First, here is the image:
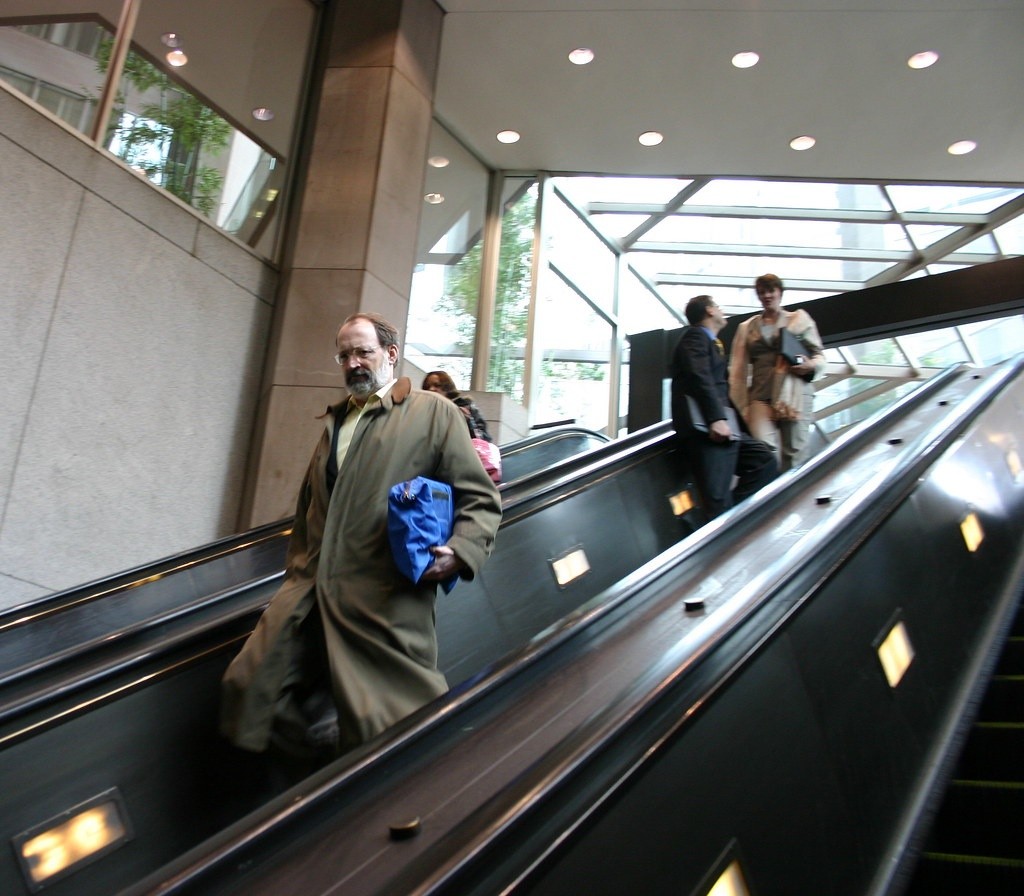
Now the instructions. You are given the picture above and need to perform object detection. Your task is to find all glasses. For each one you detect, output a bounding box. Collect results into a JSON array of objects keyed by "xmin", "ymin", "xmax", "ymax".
[
  {"xmin": 712, "ymin": 304, "xmax": 720, "ymax": 308},
  {"xmin": 335, "ymin": 345, "xmax": 382, "ymax": 366}
]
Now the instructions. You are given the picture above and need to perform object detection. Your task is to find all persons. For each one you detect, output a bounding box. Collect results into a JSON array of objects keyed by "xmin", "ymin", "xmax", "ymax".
[
  {"xmin": 223, "ymin": 314, "xmax": 503, "ymax": 873},
  {"xmin": 671, "ymin": 295, "xmax": 779, "ymax": 521},
  {"xmin": 728, "ymin": 274, "xmax": 825, "ymax": 476}
]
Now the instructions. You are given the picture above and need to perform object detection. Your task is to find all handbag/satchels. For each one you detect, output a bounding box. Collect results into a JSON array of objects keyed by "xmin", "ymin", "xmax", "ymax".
[
  {"xmin": 472, "ymin": 438, "xmax": 502, "ymax": 482},
  {"xmin": 386, "ymin": 475, "xmax": 463, "ymax": 596}
]
